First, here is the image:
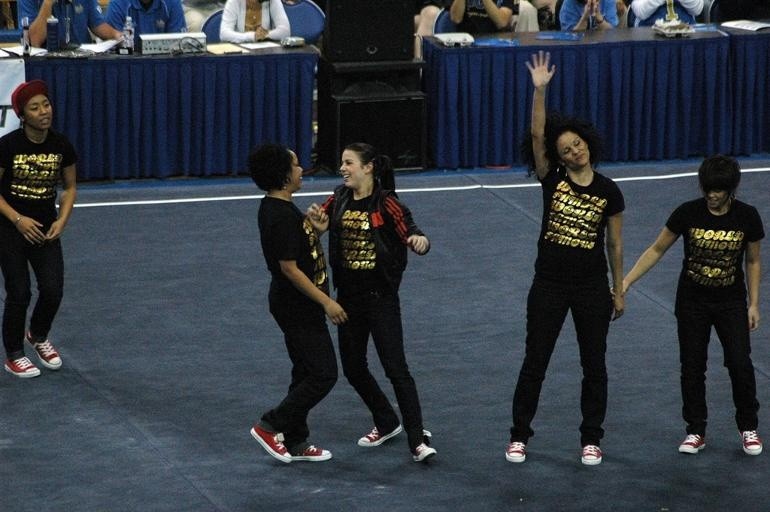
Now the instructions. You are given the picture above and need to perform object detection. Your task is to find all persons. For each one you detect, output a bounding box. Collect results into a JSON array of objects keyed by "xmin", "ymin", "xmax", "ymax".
[
  {"xmin": 0, "ymin": 80, "xmax": 79, "ymax": 380},
  {"xmin": 16, "ymin": 2, "xmax": 705, "ymax": 55},
  {"xmin": 248, "ymin": 142, "xmax": 348, "ymax": 464},
  {"xmin": 303, "ymin": 142, "xmax": 440, "ymax": 464},
  {"xmin": 608, "ymin": 153, "xmax": 765, "ymax": 457},
  {"xmin": 505, "ymin": 50, "xmax": 627, "ymax": 466}
]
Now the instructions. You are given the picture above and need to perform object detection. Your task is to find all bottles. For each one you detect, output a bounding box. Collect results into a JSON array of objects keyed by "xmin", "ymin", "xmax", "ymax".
[
  {"xmin": 123, "ymin": 15, "xmax": 136, "ymax": 53},
  {"xmin": 45, "ymin": 14, "xmax": 59, "ymax": 53}
]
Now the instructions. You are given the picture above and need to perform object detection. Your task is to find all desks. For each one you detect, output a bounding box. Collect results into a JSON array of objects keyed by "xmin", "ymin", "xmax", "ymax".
[
  {"xmin": 423, "ymin": 25, "xmax": 732, "ymax": 170},
  {"xmin": 723, "ymin": 18, "xmax": 770, "ymax": 156},
  {"xmin": 23, "ymin": 40, "xmax": 321, "ymax": 181}
]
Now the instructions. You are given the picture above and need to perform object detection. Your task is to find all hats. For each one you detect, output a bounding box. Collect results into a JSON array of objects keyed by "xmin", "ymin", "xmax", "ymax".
[{"xmin": 11, "ymin": 80, "xmax": 48, "ymax": 116}]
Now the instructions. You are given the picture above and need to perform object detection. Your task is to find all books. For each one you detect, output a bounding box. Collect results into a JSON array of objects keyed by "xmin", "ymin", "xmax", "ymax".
[{"xmin": 719, "ymin": 18, "xmax": 770, "ymax": 33}]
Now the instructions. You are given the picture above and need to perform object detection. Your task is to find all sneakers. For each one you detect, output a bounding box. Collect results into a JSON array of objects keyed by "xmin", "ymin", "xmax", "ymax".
[
  {"xmin": 4, "ymin": 357, "xmax": 41, "ymax": 378},
  {"xmin": 25, "ymin": 332, "xmax": 62, "ymax": 370},
  {"xmin": 679, "ymin": 433, "xmax": 705, "ymax": 454},
  {"xmin": 357, "ymin": 425, "xmax": 402, "ymax": 447},
  {"xmin": 249, "ymin": 427, "xmax": 293, "ymax": 464},
  {"xmin": 738, "ymin": 429, "xmax": 762, "ymax": 456},
  {"xmin": 505, "ymin": 441, "xmax": 525, "ymax": 462},
  {"xmin": 291, "ymin": 445, "xmax": 332, "ymax": 462},
  {"xmin": 412, "ymin": 443, "xmax": 437, "ymax": 461},
  {"xmin": 581, "ymin": 445, "xmax": 602, "ymax": 464}
]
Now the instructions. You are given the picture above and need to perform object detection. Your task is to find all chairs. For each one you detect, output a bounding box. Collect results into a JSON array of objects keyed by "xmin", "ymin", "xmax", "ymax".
[
  {"xmin": 413, "ymin": 4, "xmax": 442, "ymax": 78},
  {"xmin": 201, "ymin": 9, "xmax": 224, "ymax": 44},
  {"xmin": 279, "ymin": 1, "xmax": 330, "ymax": 44}
]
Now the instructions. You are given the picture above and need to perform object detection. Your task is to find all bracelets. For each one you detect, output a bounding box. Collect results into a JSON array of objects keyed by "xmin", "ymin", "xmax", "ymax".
[{"xmin": 13, "ymin": 215, "xmax": 23, "ymax": 226}]
